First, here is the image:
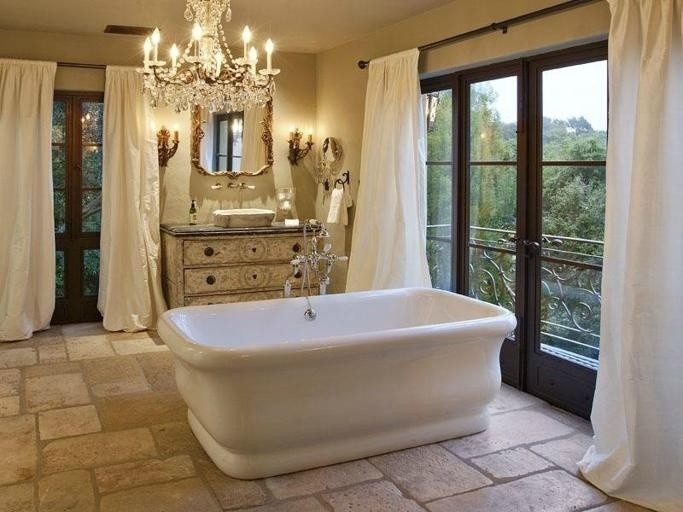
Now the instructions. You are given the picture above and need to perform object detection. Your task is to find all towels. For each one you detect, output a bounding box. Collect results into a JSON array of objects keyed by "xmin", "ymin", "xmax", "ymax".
[
  {"xmin": 326, "ymin": 188, "xmax": 349, "ymax": 227},
  {"xmin": 343, "ymin": 183, "xmax": 353, "ymax": 208}
]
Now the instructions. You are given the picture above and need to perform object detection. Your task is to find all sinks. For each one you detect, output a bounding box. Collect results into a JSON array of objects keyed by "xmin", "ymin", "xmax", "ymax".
[{"xmin": 213, "ymin": 208, "xmax": 276, "ymax": 228}]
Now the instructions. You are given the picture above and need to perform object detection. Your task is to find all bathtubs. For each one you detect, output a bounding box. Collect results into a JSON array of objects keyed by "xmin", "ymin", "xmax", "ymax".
[{"xmin": 156, "ymin": 287, "xmax": 518, "ymax": 480}]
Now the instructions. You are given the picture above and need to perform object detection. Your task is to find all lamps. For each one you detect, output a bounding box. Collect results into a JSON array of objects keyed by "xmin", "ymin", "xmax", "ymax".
[
  {"xmin": 136, "ymin": 1, "xmax": 281, "ymax": 114},
  {"xmin": 287, "ymin": 128, "xmax": 314, "ymax": 166},
  {"xmin": 155, "ymin": 124, "xmax": 179, "ymax": 167},
  {"xmin": 426, "ymin": 91, "xmax": 440, "ymax": 133}
]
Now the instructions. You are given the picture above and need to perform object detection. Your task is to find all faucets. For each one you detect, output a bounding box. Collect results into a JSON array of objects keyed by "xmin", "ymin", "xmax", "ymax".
[{"xmin": 230, "ymin": 182, "xmax": 247, "ymax": 189}]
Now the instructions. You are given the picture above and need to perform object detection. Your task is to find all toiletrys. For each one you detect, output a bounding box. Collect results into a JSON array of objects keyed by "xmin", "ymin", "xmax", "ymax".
[{"xmin": 189, "ymin": 199, "xmax": 197, "ymax": 225}]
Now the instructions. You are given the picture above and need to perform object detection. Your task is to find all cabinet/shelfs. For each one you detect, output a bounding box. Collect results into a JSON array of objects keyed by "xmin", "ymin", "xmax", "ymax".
[{"xmin": 158, "ymin": 224, "xmax": 324, "ymax": 311}]
